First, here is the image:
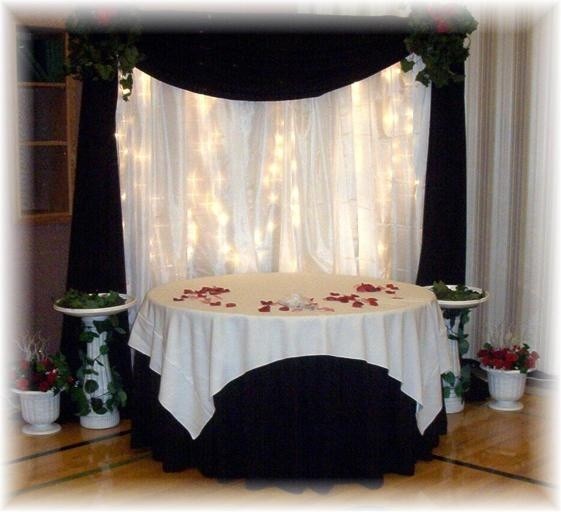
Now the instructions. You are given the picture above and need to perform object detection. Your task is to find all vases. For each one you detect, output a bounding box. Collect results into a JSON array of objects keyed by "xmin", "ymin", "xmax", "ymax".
[
  {"xmin": 12, "ymin": 389, "xmax": 65, "ymax": 435},
  {"xmin": 480, "ymin": 363, "xmax": 536, "ymax": 413}
]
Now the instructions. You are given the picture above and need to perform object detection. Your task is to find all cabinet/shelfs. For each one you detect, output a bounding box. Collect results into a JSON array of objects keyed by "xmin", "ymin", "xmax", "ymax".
[{"xmin": 8, "ymin": 17, "xmax": 77, "ymax": 224}]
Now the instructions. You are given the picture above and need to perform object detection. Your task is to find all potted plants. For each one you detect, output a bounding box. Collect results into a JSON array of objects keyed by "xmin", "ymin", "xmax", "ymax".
[
  {"xmin": 423, "ymin": 281, "xmax": 489, "ymax": 415},
  {"xmin": 51, "ymin": 288, "xmax": 136, "ymax": 430}
]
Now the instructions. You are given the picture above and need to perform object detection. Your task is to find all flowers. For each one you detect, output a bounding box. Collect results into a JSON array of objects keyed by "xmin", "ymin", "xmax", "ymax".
[
  {"xmin": 8, "ymin": 328, "xmax": 76, "ymax": 396},
  {"xmin": 474, "ymin": 338, "xmax": 539, "ymax": 377},
  {"xmin": 400, "ymin": 1, "xmax": 477, "ymax": 86},
  {"xmin": 65, "ymin": 4, "xmax": 144, "ymax": 102}
]
